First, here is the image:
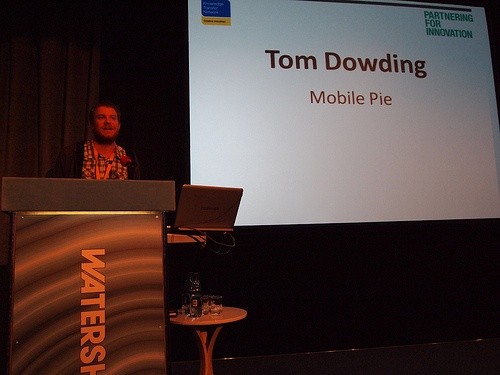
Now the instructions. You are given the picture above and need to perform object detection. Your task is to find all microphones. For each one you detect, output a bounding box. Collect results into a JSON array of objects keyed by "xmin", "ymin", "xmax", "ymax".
[{"xmin": 120, "ymin": 155, "xmax": 136, "ymax": 169}]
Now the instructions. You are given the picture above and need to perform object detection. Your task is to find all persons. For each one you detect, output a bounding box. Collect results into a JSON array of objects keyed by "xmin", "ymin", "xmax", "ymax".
[{"xmin": 47, "ymin": 99, "xmax": 154, "ymax": 180}]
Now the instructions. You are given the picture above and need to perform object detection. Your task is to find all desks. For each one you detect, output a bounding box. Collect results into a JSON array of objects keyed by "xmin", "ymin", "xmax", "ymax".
[{"xmin": 170, "ymin": 307, "xmax": 248, "ymax": 375}]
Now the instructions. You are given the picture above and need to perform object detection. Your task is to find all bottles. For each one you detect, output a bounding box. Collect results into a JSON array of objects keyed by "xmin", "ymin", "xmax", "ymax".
[
  {"xmin": 185, "ymin": 272, "xmax": 193, "ymax": 316},
  {"xmin": 189, "ymin": 272, "xmax": 202, "ymax": 318}
]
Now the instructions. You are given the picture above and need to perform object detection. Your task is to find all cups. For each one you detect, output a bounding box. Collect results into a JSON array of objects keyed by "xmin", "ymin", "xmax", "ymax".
[
  {"xmin": 211, "ymin": 295, "xmax": 223, "ymax": 314},
  {"xmin": 201, "ymin": 296, "xmax": 210, "ymax": 315}
]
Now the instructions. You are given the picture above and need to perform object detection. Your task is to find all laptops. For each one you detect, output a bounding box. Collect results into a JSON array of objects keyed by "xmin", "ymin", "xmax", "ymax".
[{"xmin": 166, "ymin": 184, "xmax": 243, "ymax": 231}]
